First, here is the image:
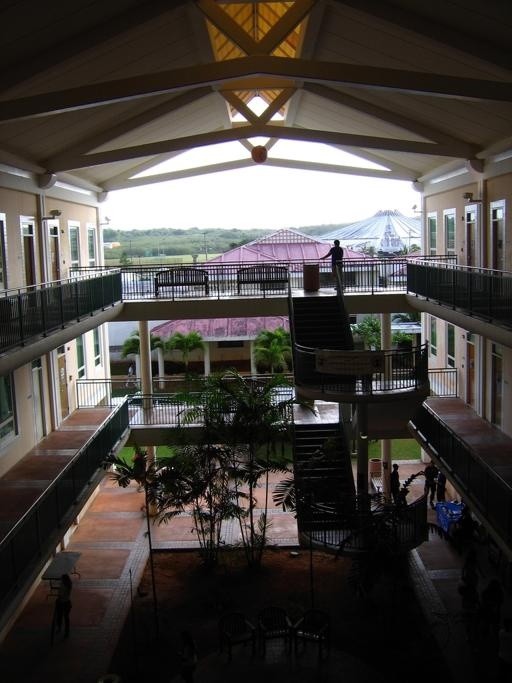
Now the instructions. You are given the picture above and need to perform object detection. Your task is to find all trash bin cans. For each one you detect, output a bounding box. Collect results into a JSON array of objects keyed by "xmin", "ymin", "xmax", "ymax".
[
  {"xmin": 370, "ymin": 459, "xmax": 381, "ymax": 477},
  {"xmin": 303, "ymin": 264, "xmax": 319, "ymax": 291}
]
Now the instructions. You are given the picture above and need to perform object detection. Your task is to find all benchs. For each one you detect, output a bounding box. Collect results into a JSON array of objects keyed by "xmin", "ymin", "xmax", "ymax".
[
  {"xmin": 154, "ymin": 266, "xmax": 291, "ymax": 295},
  {"xmin": 371, "ymin": 471, "xmax": 383, "ymax": 493}
]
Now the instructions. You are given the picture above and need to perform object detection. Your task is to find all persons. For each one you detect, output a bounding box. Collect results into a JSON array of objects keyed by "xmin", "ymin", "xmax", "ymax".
[
  {"xmin": 56, "ymin": 573, "xmax": 71, "ymax": 636},
  {"xmin": 456, "ymin": 564, "xmax": 479, "ymax": 655},
  {"xmin": 434, "ymin": 470, "xmax": 446, "ymax": 501},
  {"xmin": 479, "ymin": 575, "xmax": 505, "ymax": 640},
  {"xmin": 424, "ymin": 459, "xmax": 436, "ymax": 507},
  {"xmin": 125, "ymin": 361, "xmax": 137, "ymax": 387},
  {"xmin": 391, "ymin": 463, "xmax": 401, "ymax": 506},
  {"xmin": 319, "ymin": 239, "xmax": 344, "ymax": 289}
]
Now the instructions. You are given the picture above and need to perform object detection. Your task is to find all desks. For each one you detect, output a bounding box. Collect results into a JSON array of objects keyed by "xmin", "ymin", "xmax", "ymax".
[
  {"xmin": 43, "ymin": 550, "xmax": 84, "ymax": 599},
  {"xmin": 435, "ymin": 502, "xmax": 471, "ymax": 541}
]
